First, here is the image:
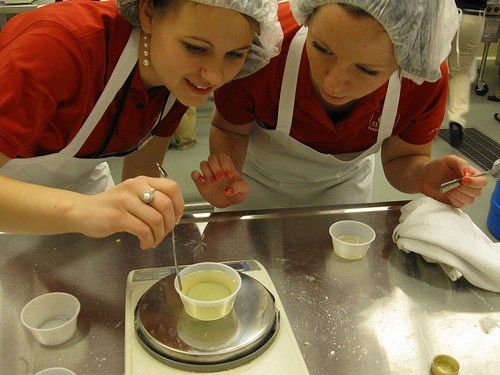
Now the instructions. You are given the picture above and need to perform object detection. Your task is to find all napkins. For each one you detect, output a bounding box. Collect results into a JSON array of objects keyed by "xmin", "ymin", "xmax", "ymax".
[{"xmin": 392, "ymin": 195, "xmax": 500, "ymax": 294}]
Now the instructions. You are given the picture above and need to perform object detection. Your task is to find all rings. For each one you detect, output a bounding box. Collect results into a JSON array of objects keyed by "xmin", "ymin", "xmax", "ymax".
[{"xmin": 142, "ymin": 188, "xmax": 156, "ymax": 203}]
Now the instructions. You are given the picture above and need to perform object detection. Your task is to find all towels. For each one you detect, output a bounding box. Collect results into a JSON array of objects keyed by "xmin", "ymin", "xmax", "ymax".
[{"xmin": 390, "ymin": 195, "xmax": 500, "ymax": 294}]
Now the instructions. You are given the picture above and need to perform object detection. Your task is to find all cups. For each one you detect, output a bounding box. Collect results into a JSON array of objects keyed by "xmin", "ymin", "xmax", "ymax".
[
  {"xmin": 20, "ymin": 291, "xmax": 81, "ymax": 346},
  {"xmin": 328, "ymin": 220, "xmax": 376, "ymax": 259},
  {"xmin": 174, "ymin": 262, "xmax": 243, "ymax": 320}
]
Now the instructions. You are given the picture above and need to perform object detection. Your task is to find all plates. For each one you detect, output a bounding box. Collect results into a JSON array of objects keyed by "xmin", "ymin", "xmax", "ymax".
[{"xmin": 33, "ymin": 366, "xmax": 76, "ymax": 375}]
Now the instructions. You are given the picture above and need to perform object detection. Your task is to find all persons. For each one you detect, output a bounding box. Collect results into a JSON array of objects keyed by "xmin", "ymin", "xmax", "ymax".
[
  {"xmin": 188, "ymin": 0, "xmax": 486, "ymax": 208},
  {"xmin": 0, "ymin": 0, "xmax": 262, "ymax": 251}
]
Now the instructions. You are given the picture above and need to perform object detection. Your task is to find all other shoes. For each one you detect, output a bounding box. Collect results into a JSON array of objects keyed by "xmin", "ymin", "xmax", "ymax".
[
  {"xmin": 449, "ymin": 122, "xmax": 463, "ymax": 147},
  {"xmin": 488, "ymin": 95, "xmax": 500, "ymax": 102}
]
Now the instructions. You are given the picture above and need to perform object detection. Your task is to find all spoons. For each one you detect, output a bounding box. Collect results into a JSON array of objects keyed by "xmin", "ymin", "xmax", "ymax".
[{"xmin": 439, "ymin": 158, "xmax": 500, "ymax": 195}]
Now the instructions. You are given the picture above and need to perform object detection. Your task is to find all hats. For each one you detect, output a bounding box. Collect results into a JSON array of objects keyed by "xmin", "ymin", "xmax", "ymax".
[
  {"xmin": 115, "ymin": 0, "xmax": 284, "ymax": 80},
  {"xmin": 288, "ymin": 0, "xmax": 459, "ymax": 85}
]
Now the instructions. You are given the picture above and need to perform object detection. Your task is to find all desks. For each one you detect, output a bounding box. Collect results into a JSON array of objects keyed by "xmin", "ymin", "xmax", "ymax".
[{"xmin": 0, "ymin": 200, "xmax": 500, "ymax": 375}]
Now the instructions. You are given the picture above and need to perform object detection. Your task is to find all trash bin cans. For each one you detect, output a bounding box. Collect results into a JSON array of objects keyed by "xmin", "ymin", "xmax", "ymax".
[{"xmin": 486, "ymin": 180, "xmax": 500, "ymax": 240}]
